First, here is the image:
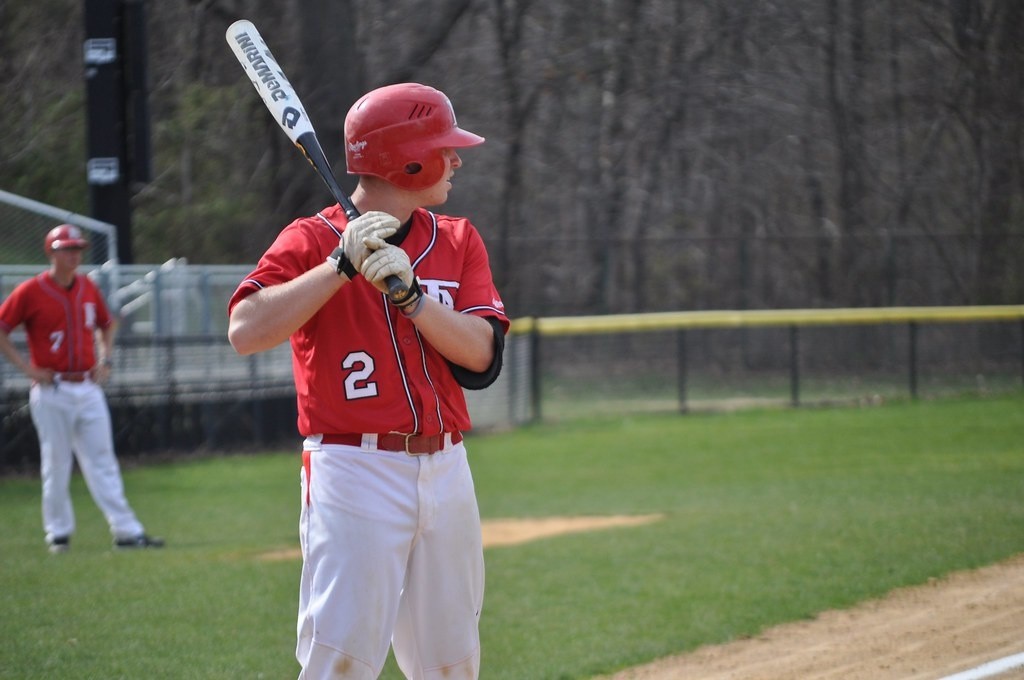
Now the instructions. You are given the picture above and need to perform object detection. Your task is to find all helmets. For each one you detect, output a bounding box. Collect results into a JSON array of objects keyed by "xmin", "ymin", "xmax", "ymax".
[
  {"xmin": 44, "ymin": 224, "xmax": 89, "ymax": 254},
  {"xmin": 344, "ymin": 82, "xmax": 485, "ymax": 192}
]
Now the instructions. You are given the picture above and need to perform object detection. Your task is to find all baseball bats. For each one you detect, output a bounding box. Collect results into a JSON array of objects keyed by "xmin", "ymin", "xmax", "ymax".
[{"xmin": 224, "ymin": 19, "xmax": 410, "ymax": 303}]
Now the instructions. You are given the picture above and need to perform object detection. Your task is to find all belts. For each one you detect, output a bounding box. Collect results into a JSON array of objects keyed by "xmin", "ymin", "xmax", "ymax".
[
  {"xmin": 321, "ymin": 430, "xmax": 463, "ymax": 456},
  {"xmin": 61, "ymin": 371, "xmax": 85, "ymax": 383}
]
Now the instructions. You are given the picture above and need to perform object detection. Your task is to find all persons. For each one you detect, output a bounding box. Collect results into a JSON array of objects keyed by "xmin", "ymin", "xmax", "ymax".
[
  {"xmin": 0, "ymin": 224, "xmax": 164, "ymax": 549},
  {"xmin": 229, "ymin": 83, "xmax": 510, "ymax": 680}
]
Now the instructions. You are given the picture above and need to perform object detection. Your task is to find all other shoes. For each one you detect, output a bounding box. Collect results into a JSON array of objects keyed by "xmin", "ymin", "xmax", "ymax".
[
  {"xmin": 49, "ymin": 535, "xmax": 69, "ymax": 552},
  {"xmin": 115, "ymin": 532, "xmax": 164, "ymax": 547}
]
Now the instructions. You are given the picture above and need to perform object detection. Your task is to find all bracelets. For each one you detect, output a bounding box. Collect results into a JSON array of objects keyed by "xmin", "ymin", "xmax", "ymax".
[
  {"xmin": 99, "ymin": 358, "xmax": 112, "ymax": 366},
  {"xmin": 400, "ymin": 294, "xmax": 425, "ymax": 318}
]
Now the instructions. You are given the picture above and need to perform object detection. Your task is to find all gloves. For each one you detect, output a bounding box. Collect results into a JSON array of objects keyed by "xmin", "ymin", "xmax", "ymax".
[
  {"xmin": 360, "ymin": 237, "xmax": 425, "ymax": 318},
  {"xmin": 326, "ymin": 210, "xmax": 400, "ymax": 282}
]
